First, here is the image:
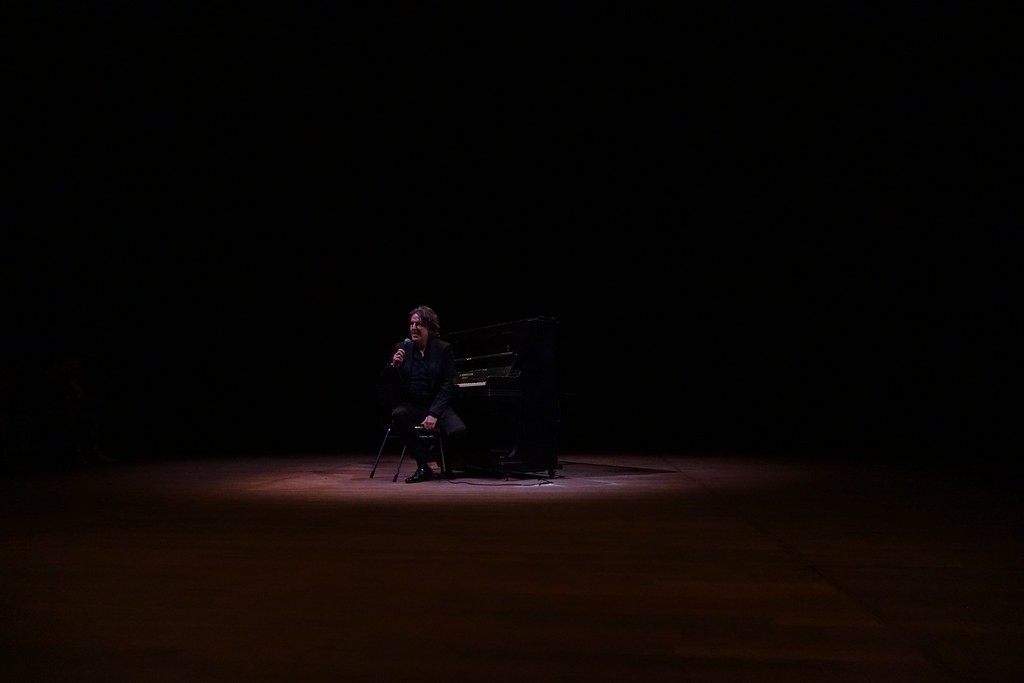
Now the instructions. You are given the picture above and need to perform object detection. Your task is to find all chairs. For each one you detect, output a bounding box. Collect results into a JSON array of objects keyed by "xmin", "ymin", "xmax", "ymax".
[{"xmin": 369, "ymin": 420, "xmax": 447, "ymax": 484}]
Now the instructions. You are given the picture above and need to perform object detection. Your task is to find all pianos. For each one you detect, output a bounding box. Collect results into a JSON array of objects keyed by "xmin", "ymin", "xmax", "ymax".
[{"xmin": 394, "ymin": 314, "xmax": 563, "ymax": 478}]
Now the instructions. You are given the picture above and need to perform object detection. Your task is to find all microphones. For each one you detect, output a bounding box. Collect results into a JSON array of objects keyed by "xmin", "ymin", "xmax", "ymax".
[{"xmin": 393, "ymin": 338, "xmax": 411, "ymax": 367}]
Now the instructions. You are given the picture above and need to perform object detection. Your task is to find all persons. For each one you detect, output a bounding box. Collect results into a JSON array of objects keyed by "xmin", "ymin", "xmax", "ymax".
[{"xmin": 378, "ymin": 306, "xmax": 467, "ymax": 483}]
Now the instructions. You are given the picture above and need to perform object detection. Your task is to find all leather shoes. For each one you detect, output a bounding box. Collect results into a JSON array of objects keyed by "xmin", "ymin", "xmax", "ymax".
[{"xmin": 405, "ymin": 468, "xmax": 433, "ymax": 483}]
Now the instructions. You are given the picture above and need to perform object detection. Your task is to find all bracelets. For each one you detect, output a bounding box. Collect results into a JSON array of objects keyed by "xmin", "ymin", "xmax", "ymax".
[{"xmin": 391, "ymin": 362, "xmax": 400, "ymax": 368}]
